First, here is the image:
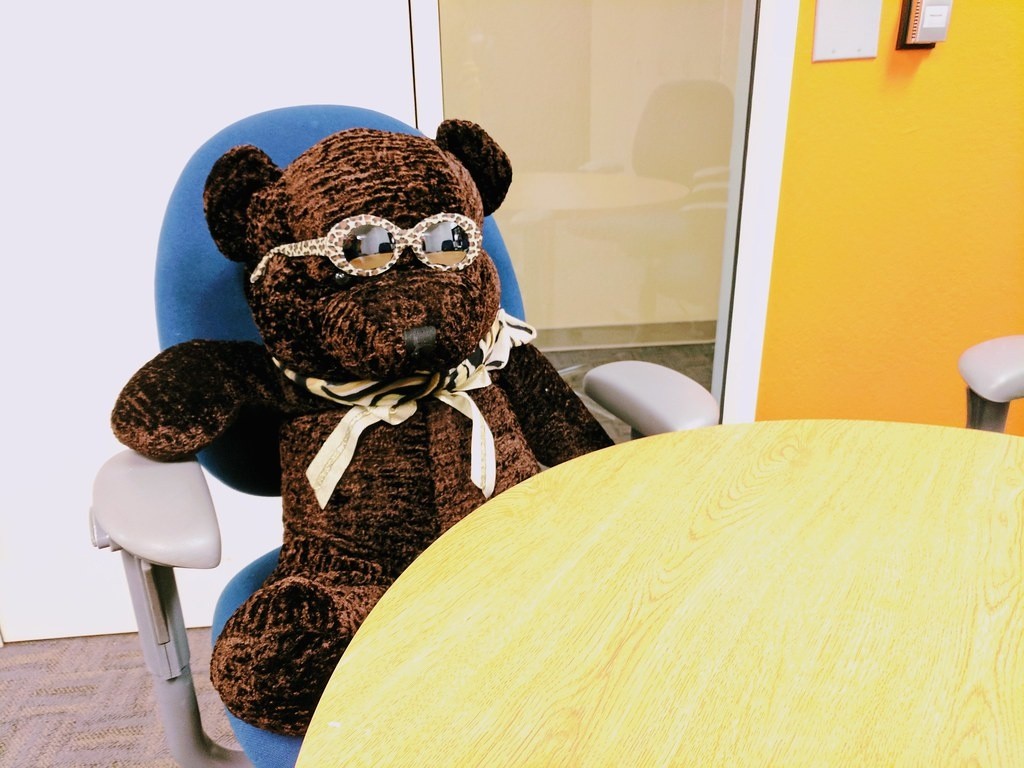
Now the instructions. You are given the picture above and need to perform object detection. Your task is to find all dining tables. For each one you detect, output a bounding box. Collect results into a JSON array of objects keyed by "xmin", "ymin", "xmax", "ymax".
[{"xmin": 295, "ymin": 418, "xmax": 1024, "ymax": 768}]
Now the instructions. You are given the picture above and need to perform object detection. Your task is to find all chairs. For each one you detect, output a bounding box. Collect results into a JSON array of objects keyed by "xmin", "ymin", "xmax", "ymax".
[{"xmin": 85, "ymin": 97, "xmax": 722, "ymax": 768}]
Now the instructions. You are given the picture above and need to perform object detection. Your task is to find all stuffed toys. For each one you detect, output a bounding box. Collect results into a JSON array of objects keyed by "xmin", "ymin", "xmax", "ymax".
[{"xmin": 108, "ymin": 118, "xmax": 616, "ymax": 740}]
